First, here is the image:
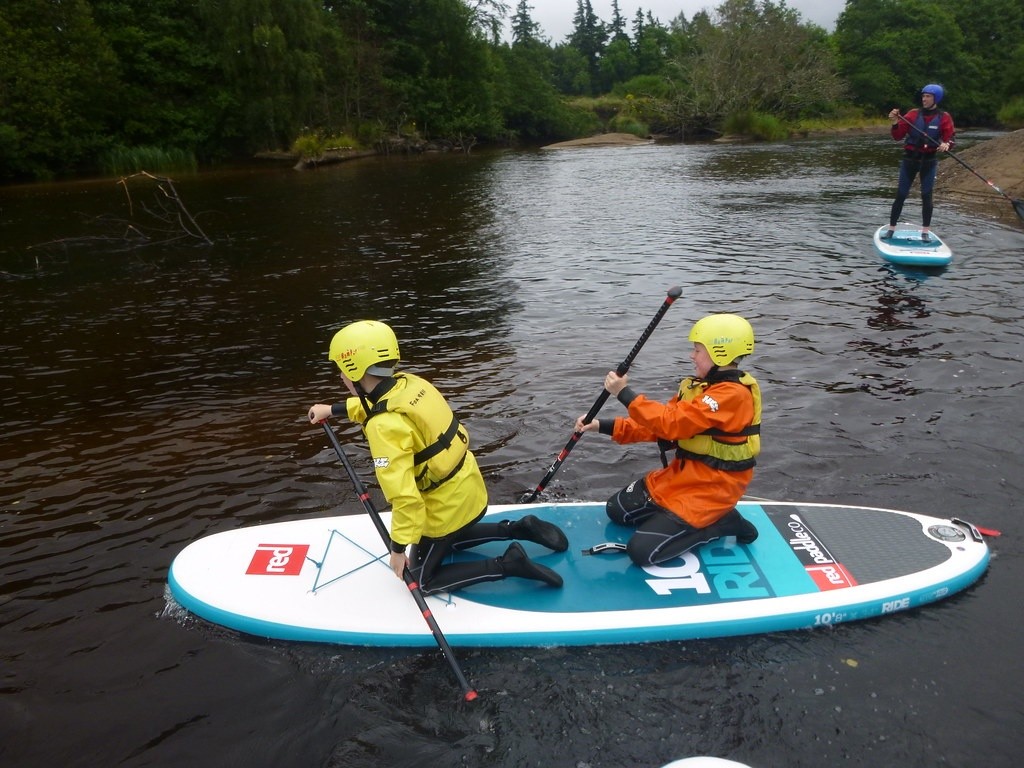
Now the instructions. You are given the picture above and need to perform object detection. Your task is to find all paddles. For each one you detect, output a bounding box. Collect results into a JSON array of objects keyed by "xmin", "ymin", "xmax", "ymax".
[
  {"xmin": 516, "ymin": 287, "xmax": 682, "ymax": 504},
  {"xmin": 892, "ymin": 109, "xmax": 1024, "ymax": 221},
  {"xmin": 312, "ymin": 408, "xmax": 506, "ymax": 749}
]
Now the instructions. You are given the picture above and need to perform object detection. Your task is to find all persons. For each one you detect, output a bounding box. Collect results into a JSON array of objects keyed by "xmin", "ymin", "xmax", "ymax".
[
  {"xmin": 308, "ymin": 320, "xmax": 569, "ymax": 597},
  {"xmin": 573, "ymin": 314, "xmax": 761, "ymax": 566},
  {"xmin": 880, "ymin": 84, "xmax": 955, "ymax": 243}
]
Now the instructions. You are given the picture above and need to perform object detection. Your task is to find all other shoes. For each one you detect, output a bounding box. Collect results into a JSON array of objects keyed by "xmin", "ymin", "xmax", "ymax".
[
  {"xmin": 495, "ymin": 542, "xmax": 563, "ymax": 587},
  {"xmin": 921, "ymin": 233, "xmax": 931, "ymax": 242},
  {"xmin": 881, "ymin": 230, "xmax": 894, "ymax": 239},
  {"xmin": 717, "ymin": 508, "xmax": 758, "ymax": 544},
  {"xmin": 498, "ymin": 515, "xmax": 568, "ymax": 552}
]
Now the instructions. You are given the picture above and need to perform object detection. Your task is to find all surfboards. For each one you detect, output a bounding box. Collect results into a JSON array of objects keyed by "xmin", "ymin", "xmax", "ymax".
[
  {"xmin": 167, "ymin": 499, "xmax": 988, "ymax": 651},
  {"xmin": 870, "ymin": 222, "xmax": 951, "ymax": 268}
]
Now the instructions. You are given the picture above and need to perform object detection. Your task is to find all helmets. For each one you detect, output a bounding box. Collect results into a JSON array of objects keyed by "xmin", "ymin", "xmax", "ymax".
[
  {"xmin": 920, "ymin": 84, "xmax": 943, "ymax": 104},
  {"xmin": 689, "ymin": 314, "xmax": 754, "ymax": 367},
  {"xmin": 329, "ymin": 320, "xmax": 401, "ymax": 382}
]
